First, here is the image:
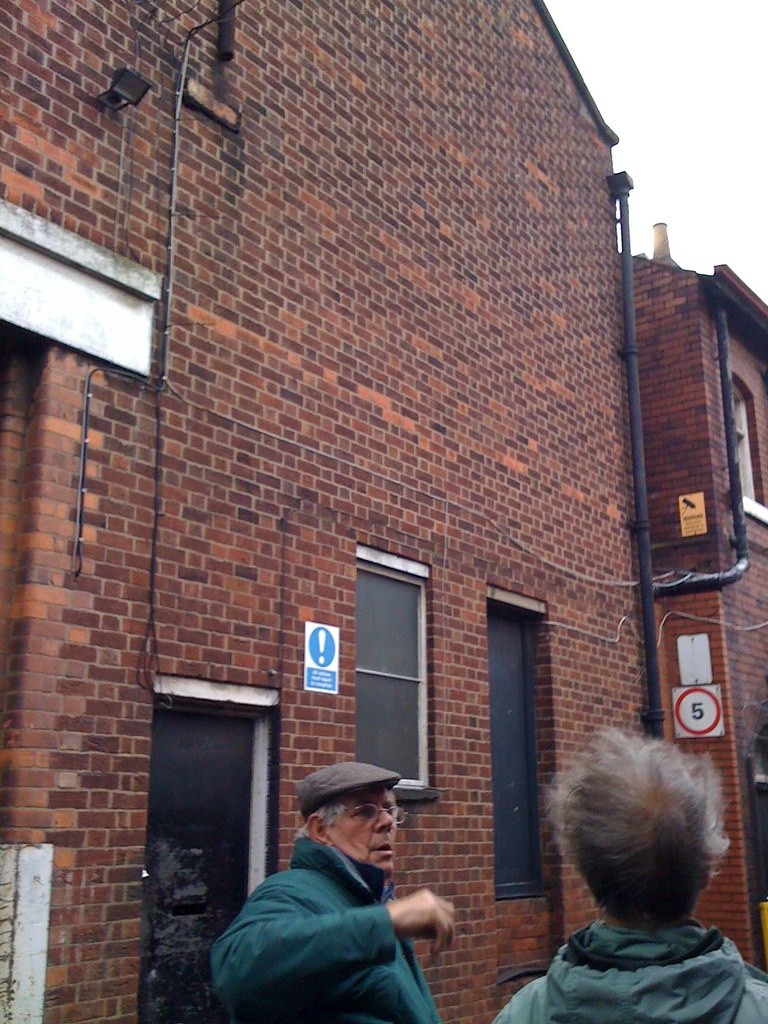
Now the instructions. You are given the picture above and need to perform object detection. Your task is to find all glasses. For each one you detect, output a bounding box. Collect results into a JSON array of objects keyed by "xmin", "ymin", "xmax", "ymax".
[{"xmin": 324, "ymin": 802, "xmax": 409, "ymax": 823}]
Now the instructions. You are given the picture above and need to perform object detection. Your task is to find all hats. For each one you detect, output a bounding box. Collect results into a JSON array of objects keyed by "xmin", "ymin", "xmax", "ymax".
[{"xmin": 298, "ymin": 762, "xmax": 402, "ymax": 822}]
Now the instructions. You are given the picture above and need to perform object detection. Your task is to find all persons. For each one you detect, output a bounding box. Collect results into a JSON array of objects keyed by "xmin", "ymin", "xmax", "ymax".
[
  {"xmin": 206, "ymin": 759, "xmax": 456, "ymax": 1024},
  {"xmin": 487, "ymin": 727, "xmax": 768, "ymax": 1023}
]
